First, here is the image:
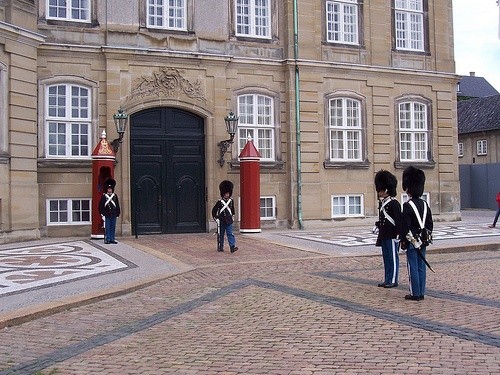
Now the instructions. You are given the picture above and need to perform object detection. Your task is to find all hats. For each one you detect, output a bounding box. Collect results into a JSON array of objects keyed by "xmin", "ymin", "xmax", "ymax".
[
  {"xmin": 402, "ymin": 166, "xmax": 425, "ymax": 197},
  {"xmin": 104, "ymin": 178, "xmax": 116, "ymax": 190},
  {"xmin": 219, "ymin": 180, "xmax": 233, "ymax": 197},
  {"xmin": 375, "ymin": 170, "xmax": 397, "ymax": 196}
]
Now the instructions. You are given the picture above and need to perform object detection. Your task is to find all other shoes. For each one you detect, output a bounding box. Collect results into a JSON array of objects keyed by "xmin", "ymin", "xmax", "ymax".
[
  {"xmin": 384, "ymin": 283, "xmax": 398, "ymax": 288},
  {"xmin": 110, "ymin": 240, "xmax": 117, "ymax": 244},
  {"xmin": 231, "ymin": 246, "xmax": 238, "ymax": 253},
  {"xmin": 220, "ymin": 248, "xmax": 224, "ymax": 252},
  {"xmin": 405, "ymin": 294, "xmax": 424, "ymax": 300},
  {"xmin": 378, "ymin": 282, "xmax": 387, "ymax": 287},
  {"xmin": 489, "ymin": 225, "xmax": 495, "ymax": 228},
  {"xmin": 105, "ymin": 241, "xmax": 109, "ymax": 244}
]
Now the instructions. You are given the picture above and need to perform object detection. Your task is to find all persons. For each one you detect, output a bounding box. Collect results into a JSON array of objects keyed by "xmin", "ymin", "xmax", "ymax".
[
  {"xmin": 372, "ymin": 170, "xmax": 402, "ymax": 288},
  {"xmin": 212, "ymin": 180, "xmax": 238, "ymax": 253},
  {"xmin": 401, "ymin": 168, "xmax": 434, "ymax": 302},
  {"xmin": 489, "ymin": 192, "xmax": 500, "ymax": 228},
  {"xmin": 97, "ymin": 178, "xmax": 120, "ymax": 244}
]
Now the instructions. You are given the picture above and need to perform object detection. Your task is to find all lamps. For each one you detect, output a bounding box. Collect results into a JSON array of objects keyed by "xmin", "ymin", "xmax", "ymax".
[
  {"xmin": 216, "ymin": 110, "xmax": 239, "ymax": 168},
  {"xmin": 109, "ymin": 106, "xmax": 129, "ymax": 167}
]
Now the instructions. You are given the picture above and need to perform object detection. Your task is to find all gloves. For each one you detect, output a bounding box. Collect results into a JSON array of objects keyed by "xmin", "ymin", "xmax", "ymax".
[
  {"xmin": 216, "ymin": 219, "xmax": 219, "ymax": 223},
  {"xmin": 372, "ymin": 226, "xmax": 380, "ymax": 234}
]
